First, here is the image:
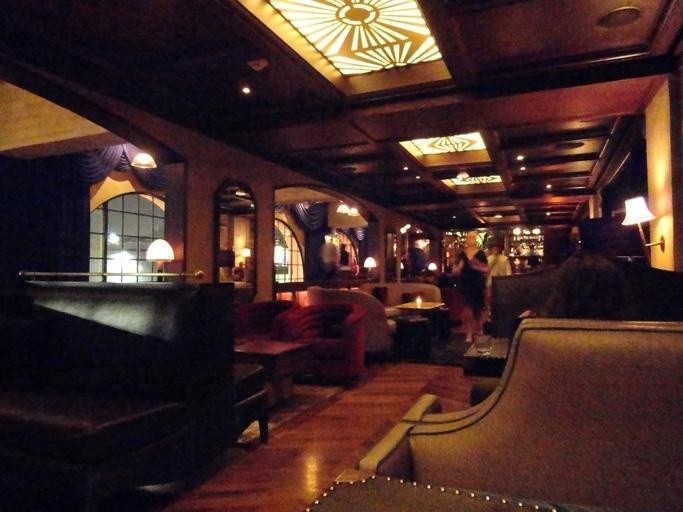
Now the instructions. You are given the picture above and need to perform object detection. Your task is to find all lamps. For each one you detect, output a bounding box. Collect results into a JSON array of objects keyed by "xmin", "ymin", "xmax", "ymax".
[
  {"xmin": 128, "ymin": 152, "xmax": 158, "ymax": 171},
  {"xmin": 620, "ymin": 196, "xmax": 665, "ymax": 252},
  {"xmin": 145, "ymin": 237, "xmax": 175, "ymax": 281}
]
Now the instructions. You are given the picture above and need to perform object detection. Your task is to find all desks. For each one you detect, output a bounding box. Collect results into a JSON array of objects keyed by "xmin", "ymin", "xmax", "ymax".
[{"xmin": 304, "ymin": 469, "xmax": 594, "ymax": 510}]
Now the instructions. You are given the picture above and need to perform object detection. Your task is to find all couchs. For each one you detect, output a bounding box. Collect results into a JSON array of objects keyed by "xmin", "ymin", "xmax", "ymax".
[{"xmin": 215, "ymin": 283, "xmax": 272, "ymax": 447}]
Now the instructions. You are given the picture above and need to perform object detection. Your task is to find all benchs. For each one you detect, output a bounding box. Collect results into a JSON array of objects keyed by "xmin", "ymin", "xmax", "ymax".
[
  {"xmin": 356, "ymin": 323, "xmax": 683, "ymax": 511},
  {"xmin": 236, "ymin": 260, "xmax": 680, "ymax": 423},
  {"xmin": 3, "ymin": 278, "xmax": 215, "ymax": 495}
]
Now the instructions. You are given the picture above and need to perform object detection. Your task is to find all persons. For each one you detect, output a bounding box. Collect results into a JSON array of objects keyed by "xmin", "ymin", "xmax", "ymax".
[
  {"xmin": 384, "ymin": 244, "xmax": 539, "ymax": 344},
  {"xmin": 562, "ymin": 234, "xmax": 634, "ymax": 321}
]
